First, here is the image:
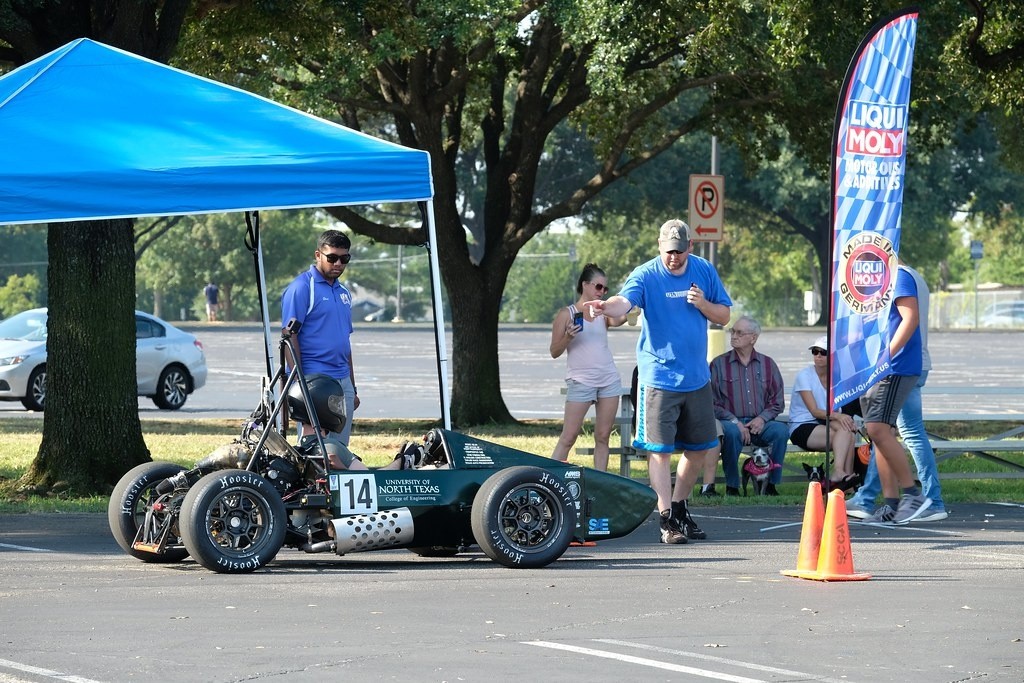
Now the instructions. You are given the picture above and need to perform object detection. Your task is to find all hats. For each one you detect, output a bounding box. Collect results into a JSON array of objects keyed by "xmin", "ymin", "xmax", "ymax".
[
  {"xmin": 659, "ymin": 220, "xmax": 692, "ymax": 253},
  {"xmin": 808, "ymin": 337, "xmax": 827, "ymax": 351}
]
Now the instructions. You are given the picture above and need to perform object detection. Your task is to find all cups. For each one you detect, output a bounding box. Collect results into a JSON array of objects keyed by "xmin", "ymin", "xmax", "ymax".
[{"xmin": 626, "ymin": 305, "xmax": 638, "ymax": 326}]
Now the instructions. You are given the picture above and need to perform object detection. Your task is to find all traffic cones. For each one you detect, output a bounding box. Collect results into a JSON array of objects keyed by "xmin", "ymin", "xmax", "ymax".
[
  {"xmin": 799, "ymin": 487, "xmax": 871, "ymax": 580},
  {"xmin": 782, "ymin": 482, "xmax": 828, "ymax": 579}
]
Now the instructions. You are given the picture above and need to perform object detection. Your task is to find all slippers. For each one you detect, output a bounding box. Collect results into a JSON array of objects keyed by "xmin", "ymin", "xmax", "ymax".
[{"xmin": 829, "ymin": 473, "xmax": 861, "ymax": 492}]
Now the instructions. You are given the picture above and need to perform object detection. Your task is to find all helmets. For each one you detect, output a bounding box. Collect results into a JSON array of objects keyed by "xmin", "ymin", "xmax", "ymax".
[{"xmin": 287, "ymin": 372, "xmax": 347, "ymax": 434}]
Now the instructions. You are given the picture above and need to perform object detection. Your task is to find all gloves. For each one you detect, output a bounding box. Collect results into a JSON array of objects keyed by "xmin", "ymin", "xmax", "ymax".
[{"xmin": 395, "ymin": 440, "xmax": 424, "ymax": 470}]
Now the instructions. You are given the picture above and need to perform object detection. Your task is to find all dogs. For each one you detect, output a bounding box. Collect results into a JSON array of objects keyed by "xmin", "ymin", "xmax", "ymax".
[
  {"xmin": 802, "ymin": 462, "xmax": 838, "ymax": 494},
  {"xmin": 741, "ymin": 443, "xmax": 774, "ymax": 496}
]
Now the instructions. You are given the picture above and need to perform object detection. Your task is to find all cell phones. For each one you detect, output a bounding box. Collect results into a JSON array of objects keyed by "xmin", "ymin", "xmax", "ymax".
[{"xmin": 574, "ymin": 312, "xmax": 583, "ymax": 331}]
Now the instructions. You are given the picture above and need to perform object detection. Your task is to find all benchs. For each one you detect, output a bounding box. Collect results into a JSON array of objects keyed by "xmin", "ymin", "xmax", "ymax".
[
  {"xmin": 624, "ymin": 442, "xmax": 866, "ymax": 454},
  {"xmin": 577, "ymin": 446, "xmax": 623, "ymax": 458}
]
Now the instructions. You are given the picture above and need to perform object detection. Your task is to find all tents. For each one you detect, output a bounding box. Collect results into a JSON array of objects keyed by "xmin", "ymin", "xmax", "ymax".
[{"xmin": 0, "ymin": 37, "xmax": 451, "ymax": 431}]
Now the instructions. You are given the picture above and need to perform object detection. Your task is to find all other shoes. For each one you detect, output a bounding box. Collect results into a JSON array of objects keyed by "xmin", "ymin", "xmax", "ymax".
[
  {"xmin": 726, "ymin": 486, "xmax": 741, "ymax": 496},
  {"xmin": 699, "ymin": 483, "xmax": 723, "ymax": 498},
  {"xmin": 767, "ymin": 484, "xmax": 780, "ymax": 497}
]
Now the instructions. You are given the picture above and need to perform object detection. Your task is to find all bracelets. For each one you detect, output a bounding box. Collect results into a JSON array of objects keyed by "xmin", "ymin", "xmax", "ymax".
[{"xmin": 354, "ymin": 387, "xmax": 358, "ymax": 395}]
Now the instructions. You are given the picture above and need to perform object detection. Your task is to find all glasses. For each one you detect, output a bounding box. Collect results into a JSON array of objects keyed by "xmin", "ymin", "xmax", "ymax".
[
  {"xmin": 812, "ymin": 349, "xmax": 827, "ymax": 357},
  {"xmin": 729, "ymin": 328, "xmax": 754, "ymax": 337},
  {"xmin": 667, "ymin": 250, "xmax": 683, "ymax": 255},
  {"xmin": 590, "ymin": 282, "xmax": 609, "ymax": 295},
  {"xmin": 319, "ymin": 250, "xmax": 351, "ymax": 265}
]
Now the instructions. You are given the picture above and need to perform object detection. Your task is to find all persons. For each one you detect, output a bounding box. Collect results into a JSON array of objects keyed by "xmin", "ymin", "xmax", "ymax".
[
  {"xmin": 282, "ymin": 229, "xmax": 360, "ymax": 447},
  {"xmin": 630, "ymin": 316, "xmax": 789, "ymax": 496},
  {"xmin": 789, "ymin": 336, "xmax": 864, "ymax": 495},
  {"xmin": 843, "ymin": 257, "xmax": 947, "ymax": 526},
  {"xmin": 582, "ymin": 220, "xmax": 734, "ymax": 544},
  {"xmin": 550, "ymin": 264, "xmax": 640, "ymax": 471},
  {"xmin": 288, "ymin": 374, "xmax": 450, "ymax": 470},
  {"xmin": 203, "ymin": 281, "xmax": 223, "ymax": 321}
]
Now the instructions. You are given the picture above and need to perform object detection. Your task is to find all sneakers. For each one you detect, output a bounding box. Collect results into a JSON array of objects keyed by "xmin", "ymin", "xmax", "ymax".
[
  {"xmin": 845, "ymin": 498, "xmax": 873, "ymax": 518},
  {"xmin": 862, "ymin": 505, "xmax": 910, "ymax": 525},
  {"xmin": 658, "ymin": 499, "xmax": 707, "ymax": 544},
  {"xmin": 892, "ymin": 493, "xmax": 933, "ymax": 523},
  {"xmin": 911, "ymin": 509, "xmax": 948, "ymax": 521}
]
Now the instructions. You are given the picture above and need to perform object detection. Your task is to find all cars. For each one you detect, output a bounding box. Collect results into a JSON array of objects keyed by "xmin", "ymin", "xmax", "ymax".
[{"xmin": 0, "ymin": 305, "xmax": 209, "ymax": 413}]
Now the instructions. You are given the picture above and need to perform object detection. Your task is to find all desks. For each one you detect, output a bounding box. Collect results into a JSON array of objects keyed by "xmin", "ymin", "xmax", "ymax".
[{"xmin": 562, "ymin": 388, "xmax": 826, "ymax": 493}]
